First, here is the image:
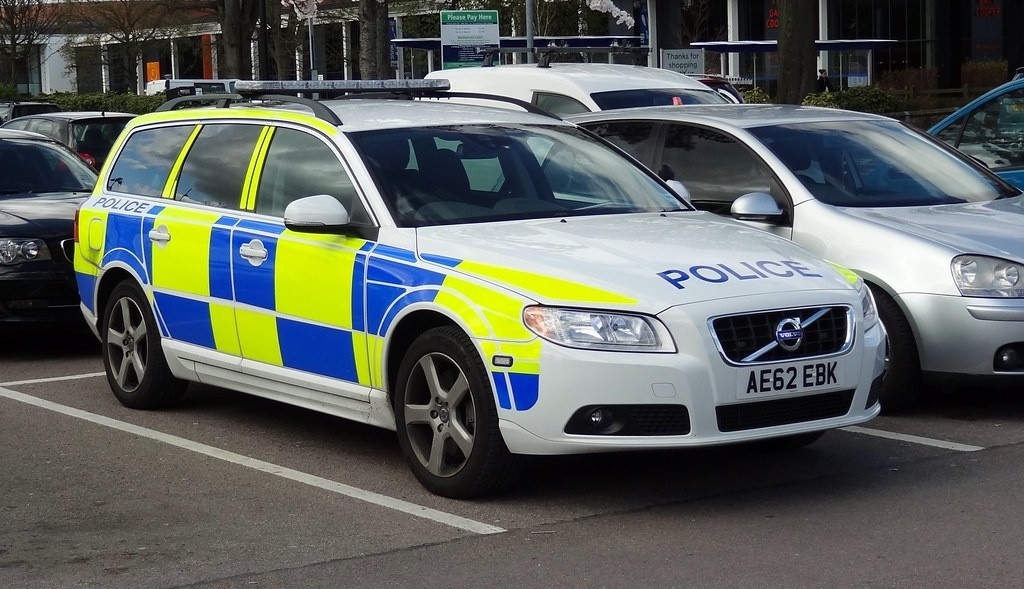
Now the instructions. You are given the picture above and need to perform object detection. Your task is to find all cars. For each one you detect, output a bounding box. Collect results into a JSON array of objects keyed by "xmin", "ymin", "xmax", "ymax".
[
  {"xmin": 875, "ymin": 67, "xmax": 1024, "ymax": 199},
  {"xmin": 1, "ymin": 111, "xmax": 142, "ymax": 177},
  {"xmin": 1, "ymin": 129, "xmax": 103, "ymax": 335}
]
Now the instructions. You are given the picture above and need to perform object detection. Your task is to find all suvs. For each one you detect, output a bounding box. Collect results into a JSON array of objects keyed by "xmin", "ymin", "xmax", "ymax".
[
  {"xmin": 71, "ymin": 86, "xmax": 892, "ymax": 498},
  {"xmin": 487, "ymin": 102, "xmax": 1024, "ymax": 404},
  {"xmin": 0, "ymin": 101, "xmax": 60, "ymax": 123},
  {"xmin": 414, "ymin": 49, "xmax": 736, "ymax": 191}
]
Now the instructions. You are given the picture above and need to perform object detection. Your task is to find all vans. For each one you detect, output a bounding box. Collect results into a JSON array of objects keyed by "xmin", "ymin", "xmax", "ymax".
[{"xmin": 144, "ymin": 80, "xmax": 242, "ymax": 94}]
